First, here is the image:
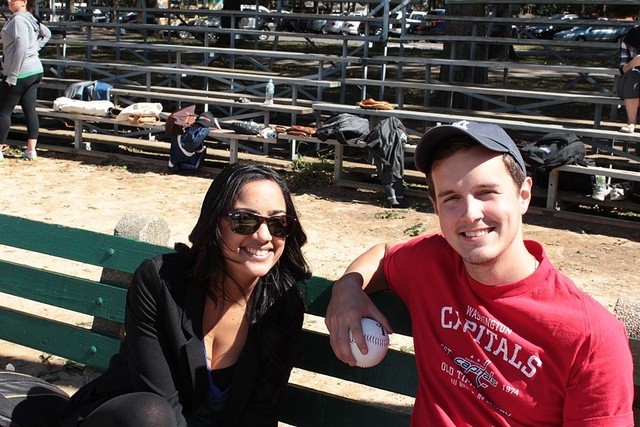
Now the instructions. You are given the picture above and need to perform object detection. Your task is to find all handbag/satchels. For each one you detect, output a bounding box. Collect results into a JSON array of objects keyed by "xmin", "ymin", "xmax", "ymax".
[{"xmin": 0, "ymin": 370, "xmax": 76, "ymax": 425}]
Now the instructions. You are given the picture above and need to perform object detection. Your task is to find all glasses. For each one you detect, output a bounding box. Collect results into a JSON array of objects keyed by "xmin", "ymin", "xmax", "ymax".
[{"xmin": 223, "ymin": 210, "xmax": 297, "ymax": 239}]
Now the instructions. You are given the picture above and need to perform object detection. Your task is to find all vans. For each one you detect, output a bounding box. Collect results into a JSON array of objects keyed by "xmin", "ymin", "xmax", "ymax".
[
  {"xmin": 548, "ymin": 13, "xmax": 580, "ymax": 19},
  {"xmin": 405, "ymin": 10, "xmax": 428, "ymax": 29}
]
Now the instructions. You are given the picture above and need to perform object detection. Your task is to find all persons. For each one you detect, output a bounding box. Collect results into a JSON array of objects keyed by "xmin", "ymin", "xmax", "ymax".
[
  {"xmin": 325, "ymin": 120, "xmax": 634, "ymax": 427},
  {"xmin": 0, "ymin": 0, "xmax": 51, "ymax": 158},
  {"xmin": 65, "ymin": 161, "xmax": 311, "ymax": 426},
  {"xmin": 616, "ymin": 24, "xmax": 640, "ymax": 133}
]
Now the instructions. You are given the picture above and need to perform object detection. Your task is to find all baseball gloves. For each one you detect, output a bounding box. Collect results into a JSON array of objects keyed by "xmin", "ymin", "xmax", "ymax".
[
  {"xmin": 285, "ymin": 126, "xmax": 317, "ymax": 136},
  {"xmin": 357, "ymin": 98, "xmax": 399, "ymax": 110}
]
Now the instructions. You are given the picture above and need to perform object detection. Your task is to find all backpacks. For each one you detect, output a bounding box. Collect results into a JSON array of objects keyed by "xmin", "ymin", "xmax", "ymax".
[
  {"xmin": 520, "ymin": 131, "xmax": 586, "ymax": 174},
  {"xmin": 316, "ymin": 113, "xmax": 370, "ymax": 145}
]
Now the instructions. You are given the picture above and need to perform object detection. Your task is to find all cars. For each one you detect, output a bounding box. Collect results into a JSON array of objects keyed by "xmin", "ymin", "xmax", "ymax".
[
  {"xmin": 553, "ymin": 17, "xmax": 625, "ymax": 43},
  {"xmin": 326, "ymin": 20, "xmax": 344, "ymax": 33},
  {"xmin": 278, "ymin": 9, "xmax": 315, "ymax": 31},
  {"xmin": 58, "ymin": 8, "xmax": 107, "ymax": 22},
  {"xmin": 341, "ymin": 20, "xmax": 361, "ymax": 34},
  {"xmin": 346, "ymin": 9, "xmax": 368, "ymax": 19},
  {"xmin": 357, "ymin": 21, "xmax": 383, "ymax": 36},
  {"xmin": 510, "ymin": 17, "xmax": 535, "ymax": 37},
  {"xmin": 103, "ymin": 10, "xmax": 161, "ymax": 35},
  {"xmin": 310, "ymin": 13, "xmax": 346, "ymax": 34},
  {"xmin": 164, "ymin": 17, "xmax": 220, "ymax": 39}
]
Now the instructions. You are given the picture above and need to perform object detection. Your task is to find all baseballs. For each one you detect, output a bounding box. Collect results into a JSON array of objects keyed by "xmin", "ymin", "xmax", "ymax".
[{"xmin": 348, "ymin": 317, "xmax": 389, "ymax": 369}]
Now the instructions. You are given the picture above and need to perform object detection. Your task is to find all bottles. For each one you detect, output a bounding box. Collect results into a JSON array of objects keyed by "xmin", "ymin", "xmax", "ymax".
[{"xmin": 265, "ymin": 79, "xmax": 275, "ymax": 105}]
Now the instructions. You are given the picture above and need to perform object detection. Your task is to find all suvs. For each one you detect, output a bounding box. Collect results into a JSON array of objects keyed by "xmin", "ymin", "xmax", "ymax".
[
  {"xmin": 533, "ymin": 17, "xmax": 573, "ymax": 40},
  {"xmin": 420, "ymin": 9, "xmax": 447, "ymax": 42},
  {"xmin": 193, "ymin": 4, "xmax": 277, "ymax": 41},
  {"xmin": 388, "ymin": 10, "xmax": 410, "ymax": 34}
]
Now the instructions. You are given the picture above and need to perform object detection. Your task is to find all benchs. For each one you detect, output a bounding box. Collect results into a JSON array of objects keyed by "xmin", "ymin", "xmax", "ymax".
[
  {"xmin": 43, "ymin": 39, "xmax": 361, "ymax": 81},
  {"xmin": 32, "ymin": 77, "xmax": 313, "ymax": 163},
  {"xmin": 0, "ymin": 104, "xmax": 277, "ymax": 175},
  {"xmin": 1, "ymin": 213, "xmax": 639, "ymax": 427},
  {"xmin": 312, "ymin": 100, "xmax": 640, "ymax": 154},
  {"xmin": 36, "ymin": 58, "xmax": 342, "ymax": 127},
  {"xmin": 367, "ymin": 55, "xmax": 622, "ymax": 99},
  {"xmin": 422, "ymin": 12, "xmax": 640, "ymax": 110},
  {"xmin": 398, "ymin": 34, "xmax": 620, "ymax": 106},
  {"xmin": 337, "ymin": 77, "xmax": 640, "ymax": 154},
  {"xmin": 38, "ymin": 7, "xmax": 399, "ymax": 37},
  {"xmin": 42, "ymin": 21, "xmax": 382, "ymax": 58}
]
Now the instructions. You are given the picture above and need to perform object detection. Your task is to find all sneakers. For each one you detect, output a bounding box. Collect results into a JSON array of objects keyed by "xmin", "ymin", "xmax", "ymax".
[
  {"xmin": 610, "ymin": 182, "xmax": 627, "ymax": 202},
  {"xmin": 22, "ymin": 150, "xmax": 37, "ymax": 159},
  {"xmin": 619, "ymin": 126, "xmax": 635, "ymax": 133},
  {"xmin": 590, "ymin": 181, "xmax": 613, "ymax": 202},
  {"xmin": 0, "ymin": 151, "xmax": 5, "ymax": 162}
]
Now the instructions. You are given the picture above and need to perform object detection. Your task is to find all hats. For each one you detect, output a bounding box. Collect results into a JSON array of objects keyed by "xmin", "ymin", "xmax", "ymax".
[
  {"xmin": 413, "ymin": 120, "xmax": 527, "ymax": 176},
  {"xmin": 193, "ymin": 112, "xmax": 214, "ymax": 128}
]
